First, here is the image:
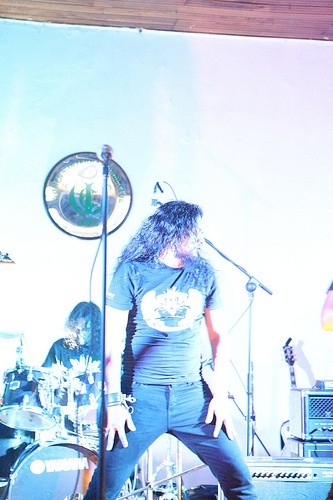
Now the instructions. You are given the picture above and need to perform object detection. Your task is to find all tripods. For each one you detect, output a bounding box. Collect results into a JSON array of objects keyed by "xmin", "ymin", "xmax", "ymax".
[{"xmin": 139, "ymin": 434, "xmax": 187, "ymax": 500}]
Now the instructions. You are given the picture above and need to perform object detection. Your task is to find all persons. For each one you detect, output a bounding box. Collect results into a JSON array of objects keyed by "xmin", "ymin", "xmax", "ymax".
[
  {"xmin": 40, "ymin": 300, "xmax": 101, "ymax": 440},
  {"xmin": 82, "ymin": 201, "xmax": 260, "ymax": 500}
]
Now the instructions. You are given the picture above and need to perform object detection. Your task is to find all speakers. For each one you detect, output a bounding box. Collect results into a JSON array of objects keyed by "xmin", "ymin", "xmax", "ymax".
[{"xmin": 217, "ymin": 380, "xmax": 333, "ymax": 500}]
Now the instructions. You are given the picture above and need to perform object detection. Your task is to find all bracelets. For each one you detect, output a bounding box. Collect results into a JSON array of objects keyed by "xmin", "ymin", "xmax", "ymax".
[{"xmin": 103, "ymin": 392, "xmax": 124, "ymax": 407}]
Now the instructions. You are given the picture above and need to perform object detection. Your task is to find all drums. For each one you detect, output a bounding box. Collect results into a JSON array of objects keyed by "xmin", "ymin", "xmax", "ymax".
[
  {"xmin": 67, "ymin": 370, "xmax": 105, "ymax": 423},
  {"xmin": 0, "ymin": 366, "xmax": 62, "ymax": 431},
  {"xmin": 4, "ymin": 438, "xmax": 125, "ymax": 500}
]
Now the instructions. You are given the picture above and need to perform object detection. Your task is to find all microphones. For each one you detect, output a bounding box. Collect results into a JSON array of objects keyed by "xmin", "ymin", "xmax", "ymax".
[
  {"xmin": 148, "ymin": 182, "xmax": 160, "ymax": 214},
  {"xmin": 97, "ymin": 144, "xmax": 114, "ymax": 163}
]
